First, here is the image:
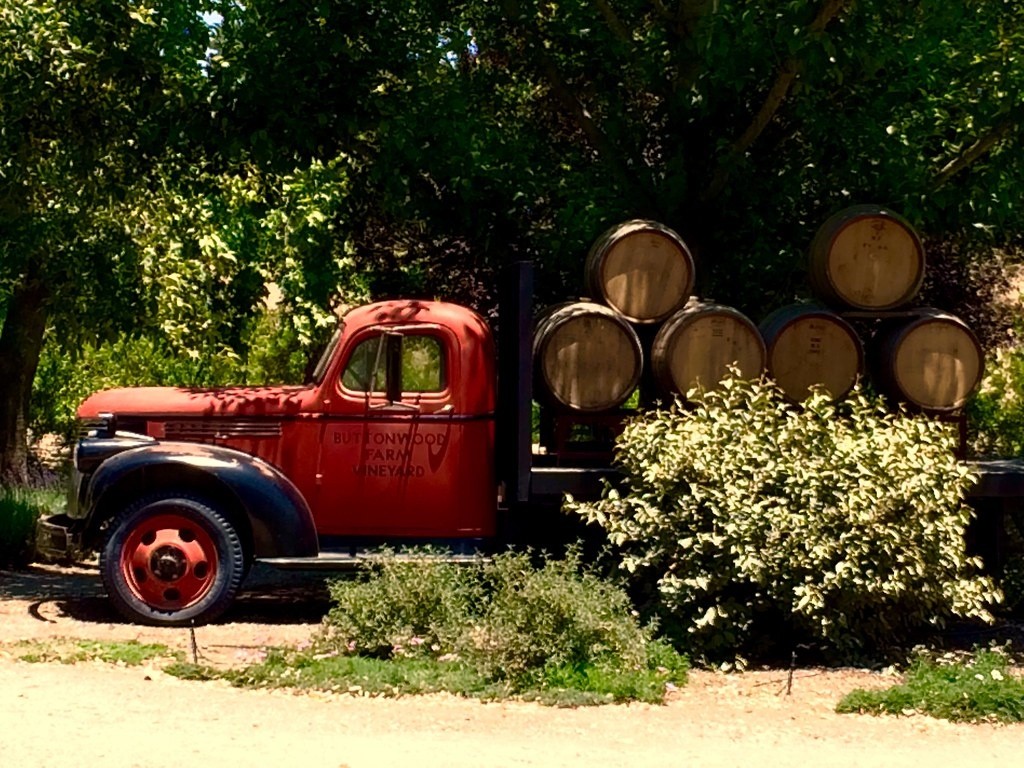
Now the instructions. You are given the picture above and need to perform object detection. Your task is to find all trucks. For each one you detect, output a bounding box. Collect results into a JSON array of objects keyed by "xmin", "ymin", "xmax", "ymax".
[{"xmin": 33, "ymin": 261, "xmax": 1024, "ymax": 631}]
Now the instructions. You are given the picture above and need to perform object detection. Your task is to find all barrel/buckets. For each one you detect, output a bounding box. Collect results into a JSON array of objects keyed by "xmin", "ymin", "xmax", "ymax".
[
  {"xmin": 866, "ymin": 308, "xmax": 986, "ymax": 417},
  {"xmin": 529, "ymin": 294, "xmax": 644, "ymax": 415},
  {"xmin": 759, "ymin": 303, "xmax": 865, "ymax": 414},
  {"xmin": 583, "ymin": 219, "xmax": 696, "ymax": 340},
  {"xmin": 637, "ymin": 294, "xmax": 768, "ymax": 413},
  {"xmin": 807, "ymin": 202, "xmax": 926, "ymax": 312}
]
